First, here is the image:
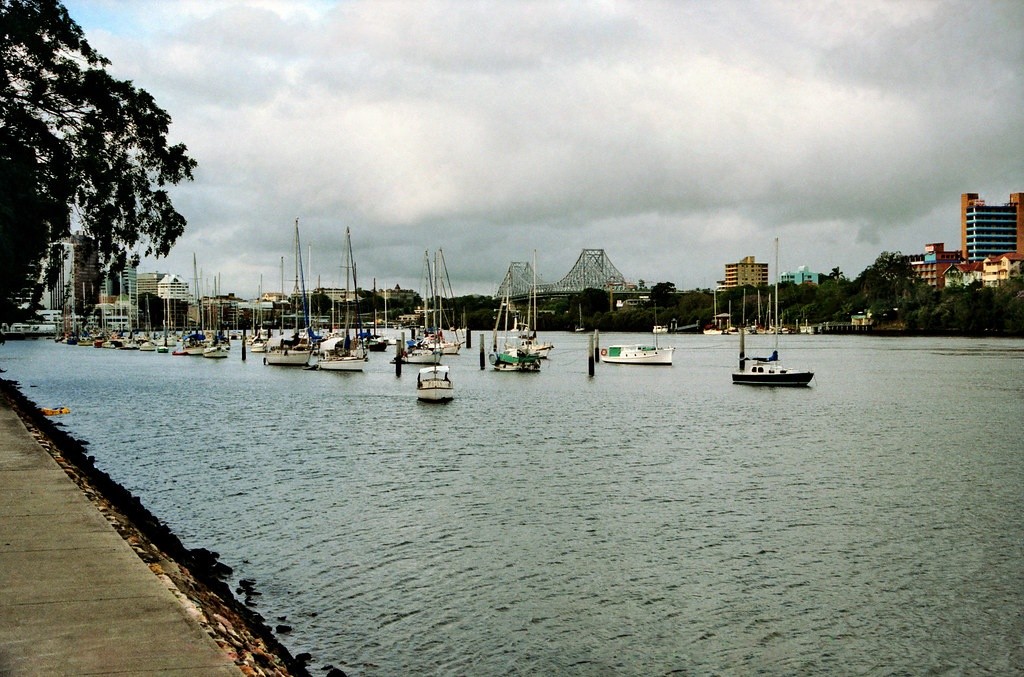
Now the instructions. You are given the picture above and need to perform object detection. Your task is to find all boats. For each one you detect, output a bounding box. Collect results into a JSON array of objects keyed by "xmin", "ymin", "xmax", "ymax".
[{"xmin": 40, "ymin": 404, "xmax": 74, "ymax": 419}]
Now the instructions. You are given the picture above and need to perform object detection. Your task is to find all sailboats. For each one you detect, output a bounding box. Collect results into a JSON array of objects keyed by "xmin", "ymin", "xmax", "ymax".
[{"xmin": 53, "ymin": 219, "xmax": 814, "ymax": 404}]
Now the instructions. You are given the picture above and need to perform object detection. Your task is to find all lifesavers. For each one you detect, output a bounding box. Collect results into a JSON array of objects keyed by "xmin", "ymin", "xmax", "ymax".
[
  {"xmin": 43, "ymin": 407, "xmax": 70, "ymax": 415},
  {"xmin": 601, "ymin": 349, "xmax": 607, "ymax": 355}
]
